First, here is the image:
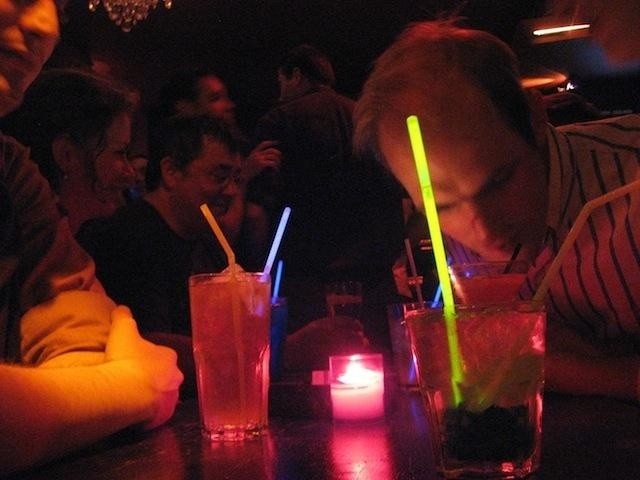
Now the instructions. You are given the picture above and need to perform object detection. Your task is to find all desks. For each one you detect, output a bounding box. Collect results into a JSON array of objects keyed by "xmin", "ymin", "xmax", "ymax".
[{"xmin": 2, "ymin": 364, "xmax": 639, "ymax": 480}]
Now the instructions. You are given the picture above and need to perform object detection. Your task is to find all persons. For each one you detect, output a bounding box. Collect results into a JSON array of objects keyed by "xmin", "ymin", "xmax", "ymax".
[
  {"xmin": 0, "ymin": 45, "xmax": 404, "ymax": 399},
  {"xmin": 351, "ymin": 16, "xmax": 640, "ymax": 399},
  {"xmin": 0, "ymin": 0, "xmax": 188, "ymax": 479}
]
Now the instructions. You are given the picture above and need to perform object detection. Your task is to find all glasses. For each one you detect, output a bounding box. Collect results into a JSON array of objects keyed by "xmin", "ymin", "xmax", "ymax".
[{"xmin": 213, "ymin": 175, "xmax": 245, "ymax": 186}]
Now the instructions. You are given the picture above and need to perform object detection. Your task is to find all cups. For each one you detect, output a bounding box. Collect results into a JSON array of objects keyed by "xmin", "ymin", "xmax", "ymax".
[
  {"xmin": 448, "ymin": 260, "xmax": 531, "ymax": 311},
  {"xmin": 385, "ymin": 302, "xmax": 419, "ymax": 393},
  {"xmin": 325, "ymin": 353, "xmax": 385, "ymax": 422},
  {"xmin": 188, "ymin": 271, "xmax": 273, "ymax": 440},
  {"xmin": 269, "ymin": 298, "xmax": 292, "ymax": 417},
  {"xmin": 320, "ymin": 281, "xmax": 365, "ymax": 324},
  {"xmin": 403, "ymin": 301, "xmax": 550, "ymax": 473}
]
